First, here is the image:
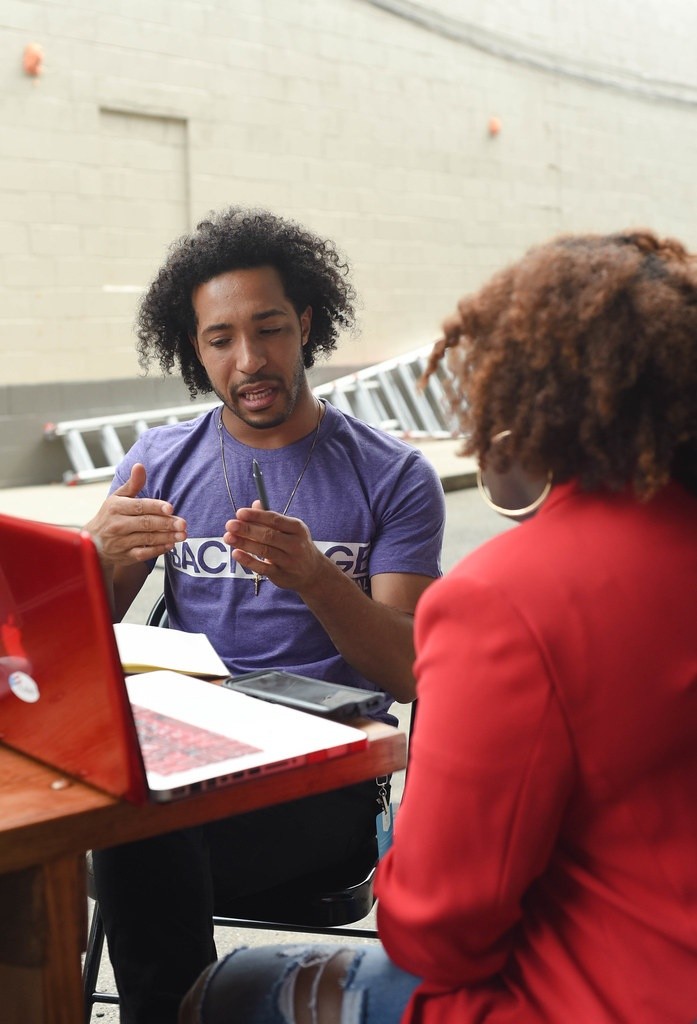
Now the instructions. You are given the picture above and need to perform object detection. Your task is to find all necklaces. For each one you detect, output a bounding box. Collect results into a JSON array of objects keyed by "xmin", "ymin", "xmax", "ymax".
[{"xmin": 217, "ymin": 394, "xmax": 323, "ymax": 596}]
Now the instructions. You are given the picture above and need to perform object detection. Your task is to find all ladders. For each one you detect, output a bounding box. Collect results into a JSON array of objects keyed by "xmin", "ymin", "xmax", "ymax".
[
  {"xmin": 330, "ymin": 345, "xmax": 480, "ymax": 441},
  {"xmin": 40, "ymin": 381, "xmax": 404, "ymax": 485}
]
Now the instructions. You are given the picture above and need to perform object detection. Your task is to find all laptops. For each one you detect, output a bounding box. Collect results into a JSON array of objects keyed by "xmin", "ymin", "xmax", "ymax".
[{"xmin": 0, "ymin": 514, "xmax": 370, "ymax": 805}]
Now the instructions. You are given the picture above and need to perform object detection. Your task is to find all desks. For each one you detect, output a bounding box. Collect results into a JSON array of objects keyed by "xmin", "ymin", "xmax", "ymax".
[{"xmin": 0, "ymin": 672, "xmax": 408, "ymax": 1024}]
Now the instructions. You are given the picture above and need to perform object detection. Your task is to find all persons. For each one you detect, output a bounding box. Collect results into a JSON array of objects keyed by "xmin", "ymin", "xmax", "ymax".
[
  {"xmin": 171, "ymin": 233, "xmax": 697, "ymax": 1024},
  {"xmin": 77, "ymin": 203, "xmax": 446, "ymax": 1024}
]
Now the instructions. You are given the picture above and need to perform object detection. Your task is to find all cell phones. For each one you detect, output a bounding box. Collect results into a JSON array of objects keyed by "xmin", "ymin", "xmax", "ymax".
[{"xmin": 221, "ymin": 670, "xmax": 386, "ymax": 720}]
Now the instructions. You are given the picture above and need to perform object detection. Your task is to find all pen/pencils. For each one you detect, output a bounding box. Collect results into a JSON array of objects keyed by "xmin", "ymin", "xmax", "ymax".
[{"xmin": 252, "ymin": 459, "xmax": 272, "ymax": 510}]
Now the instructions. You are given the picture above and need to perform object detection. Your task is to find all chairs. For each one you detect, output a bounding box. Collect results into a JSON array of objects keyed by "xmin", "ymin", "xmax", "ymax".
[{"xmin": 81, "ymin": 698, "xmax": 420, "ymax": 1024}]
